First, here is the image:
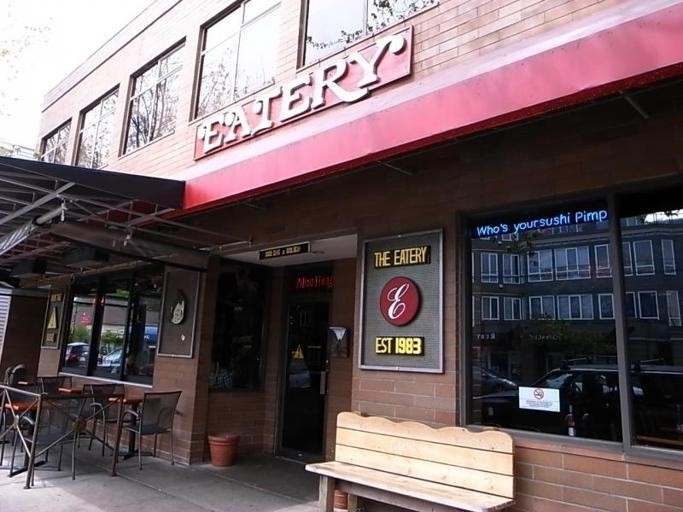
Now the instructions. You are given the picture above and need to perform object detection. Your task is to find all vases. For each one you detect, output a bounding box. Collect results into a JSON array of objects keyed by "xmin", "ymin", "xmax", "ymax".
[
  {"xmin": 334, "ymin": 489, "xmax": 346, "ymax": 509},
  {"xmin": 206, "ymin": 432, "xmax": 241, "ymax": 468}
]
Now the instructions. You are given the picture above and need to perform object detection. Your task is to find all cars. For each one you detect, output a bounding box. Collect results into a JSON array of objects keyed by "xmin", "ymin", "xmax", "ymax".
[
  {"xmin": 468, "ymin": 365, "xmax": 525, "ymax": 422},
  {"xmin": 62, "ymin": 322, "xmax": 158, "ymax": 377}
]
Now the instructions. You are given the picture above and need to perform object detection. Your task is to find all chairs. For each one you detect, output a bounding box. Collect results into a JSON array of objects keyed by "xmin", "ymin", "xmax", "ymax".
[{"xmin": 0, "ymin": 361, "xmax": 181, "ymax": 495}]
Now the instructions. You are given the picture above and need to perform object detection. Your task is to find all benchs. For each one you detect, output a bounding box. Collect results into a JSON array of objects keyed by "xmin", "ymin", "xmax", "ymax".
[{"xmin": 304, "ymin": 405, "xmax": 517, "ymax": 512}]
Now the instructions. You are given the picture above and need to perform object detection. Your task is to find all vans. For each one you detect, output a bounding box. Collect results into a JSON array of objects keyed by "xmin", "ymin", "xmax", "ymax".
[{"xmin": 525, "ymin": 353, "xmax": 683, "ymax": 449}]
